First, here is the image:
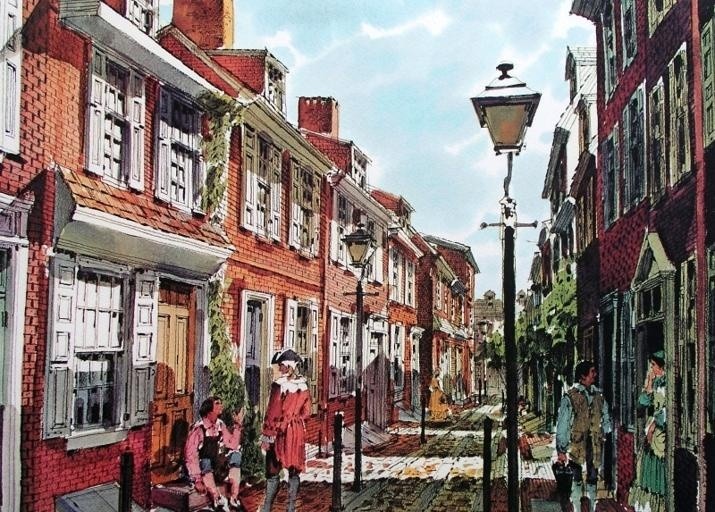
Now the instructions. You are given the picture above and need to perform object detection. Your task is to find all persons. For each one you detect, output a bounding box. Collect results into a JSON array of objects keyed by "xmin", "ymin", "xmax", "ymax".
[
  {"xmin": 556, "ymin": 362, "xmax": 612, "ymax": 512},
  {"xmin": 183, "ymin": 395, "xmax": 248, "ymax": 510},
  {"xmin": 256, "ymin": 349, "xmax": 313, "ymax": 511},
  {"xmin": 627, "ymin": 349, "xmax": 668, "ymax": 512},
  {"xmin": 428, "ymin": 368, "xmax": 450, "ymax": 420}
]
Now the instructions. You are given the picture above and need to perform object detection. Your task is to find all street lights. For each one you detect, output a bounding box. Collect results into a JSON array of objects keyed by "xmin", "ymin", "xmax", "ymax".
[
  {"xmin": 470, "ymin": 59, "xmax": 541, "ymax": 511},
  {"xmin": 474, "ymin": 315, "xmax": 493, "ymax": 403},
  {"xmin": 340, "ymin": 220, "xmax": 381, "ymax": 492}
]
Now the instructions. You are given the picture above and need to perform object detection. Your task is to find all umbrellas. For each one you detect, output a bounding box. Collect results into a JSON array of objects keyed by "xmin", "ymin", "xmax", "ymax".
[{"xmin": 551, "ymin": 458, "xmax": 574, "ymax": 512}]
